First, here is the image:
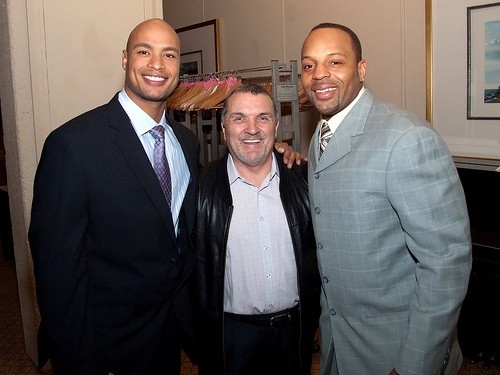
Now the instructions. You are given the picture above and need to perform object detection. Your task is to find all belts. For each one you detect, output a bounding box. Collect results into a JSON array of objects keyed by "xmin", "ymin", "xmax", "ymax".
[{"xmin": 224, "ymin": 306, "xmax": 300, "ymax": 329}]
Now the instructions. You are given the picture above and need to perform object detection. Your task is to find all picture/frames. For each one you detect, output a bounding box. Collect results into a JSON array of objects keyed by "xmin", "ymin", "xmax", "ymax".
[
  {"xmin": 426, "ymin": 0, "xmax": 500, "ymax": 169},
  {"xmin": 173, "ymin": 18, "xmax": 222, "ymax": 145}
]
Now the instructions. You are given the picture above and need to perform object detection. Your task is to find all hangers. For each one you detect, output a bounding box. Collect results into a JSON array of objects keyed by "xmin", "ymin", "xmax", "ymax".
[{"xmin": 168, "ymin": 70, "xmax": 313, "ymax": 111}]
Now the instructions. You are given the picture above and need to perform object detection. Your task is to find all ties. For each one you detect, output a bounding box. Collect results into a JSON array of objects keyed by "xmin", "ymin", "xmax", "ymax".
[
  {"xmin": 149, "ymin": 125, "xmax": 171, "ymax": 210},
  {"xmin": 319, "ymin": 122, "xmax": 333, "ymax": 160}
]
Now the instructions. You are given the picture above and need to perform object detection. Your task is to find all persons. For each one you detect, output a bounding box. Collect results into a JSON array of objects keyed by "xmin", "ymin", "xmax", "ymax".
[
  {"xmin": 301, "ymin": 22, "xmax": 473, "ymax": 375},
  {"xmin": 195, "ymin": 85, "xmax": 322, "ymax": 375},
  {"xmin": 28, "ymin": 19, "xmax": 308, "ymax": 375}
]
